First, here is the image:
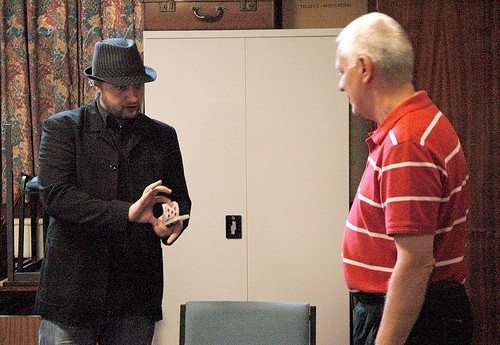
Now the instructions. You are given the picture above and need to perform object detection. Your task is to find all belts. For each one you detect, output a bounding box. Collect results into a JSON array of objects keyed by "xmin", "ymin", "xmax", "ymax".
[{"xmin": 353, "ymin": 280, "xmax": 460, "ymax": 306}]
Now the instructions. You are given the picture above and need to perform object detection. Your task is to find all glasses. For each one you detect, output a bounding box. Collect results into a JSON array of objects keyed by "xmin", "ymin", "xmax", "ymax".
[{"xmin": 337, "ymin": 61, "xmax": 359, "ymax": 79}]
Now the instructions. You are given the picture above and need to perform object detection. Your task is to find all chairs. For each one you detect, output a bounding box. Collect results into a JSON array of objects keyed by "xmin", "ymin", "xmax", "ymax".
[{"xmin": 179, "ymin": 301, "xmax": 316, "ymax": 345}]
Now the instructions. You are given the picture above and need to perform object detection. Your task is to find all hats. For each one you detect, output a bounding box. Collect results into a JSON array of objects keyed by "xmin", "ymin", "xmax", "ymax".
[{"xmin": 84, "ymin": 38, "xmax": 157, "ymax": 86}]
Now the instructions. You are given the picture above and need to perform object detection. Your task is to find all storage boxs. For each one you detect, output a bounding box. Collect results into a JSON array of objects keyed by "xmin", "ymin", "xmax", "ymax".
[{"xmin": 281, "ymin": 0, "xmax": 368, "ymax": 29}]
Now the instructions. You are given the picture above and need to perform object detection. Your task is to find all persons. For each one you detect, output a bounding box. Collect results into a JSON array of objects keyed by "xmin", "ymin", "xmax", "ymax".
[
  {"xmin": 35, "ymin": 38, "xmax": 192, "ymax": 345},
  {"xmin": 335, "ymin": 12, "xmax": 473, "ymax": 345}
]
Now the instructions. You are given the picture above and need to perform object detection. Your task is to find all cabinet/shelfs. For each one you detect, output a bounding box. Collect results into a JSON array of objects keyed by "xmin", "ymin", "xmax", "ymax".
[{"xmin": 142, "ymin": 29, "xmax": 350, "ymax": 345}]
